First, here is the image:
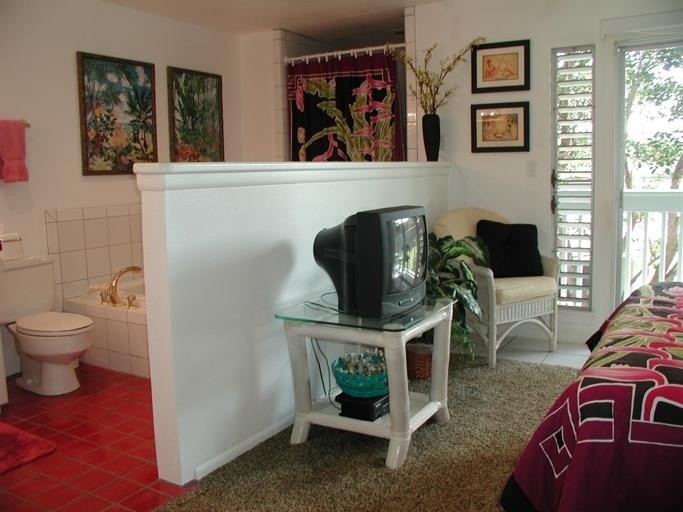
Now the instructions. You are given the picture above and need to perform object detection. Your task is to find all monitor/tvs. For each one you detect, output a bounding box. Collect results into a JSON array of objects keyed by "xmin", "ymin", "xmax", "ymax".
[{"xmin": 313, "ymin": 205, "xmax": 430, "ymax": 320}]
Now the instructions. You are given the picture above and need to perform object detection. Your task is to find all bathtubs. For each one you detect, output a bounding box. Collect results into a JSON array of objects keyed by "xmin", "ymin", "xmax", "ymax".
[{"xmin": 63, "ymin": 280, "xmax": 149, "ymax": 377}]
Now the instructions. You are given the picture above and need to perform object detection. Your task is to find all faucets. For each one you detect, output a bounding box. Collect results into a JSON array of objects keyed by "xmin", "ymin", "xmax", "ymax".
[{"xmin": 109, "ymin": 266, "xmax": 141, "ymax": 307}]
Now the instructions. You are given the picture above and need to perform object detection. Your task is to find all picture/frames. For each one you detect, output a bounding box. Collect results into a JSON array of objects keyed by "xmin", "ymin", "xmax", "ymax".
[
  {"xmin": 167, "ymin": 66, "xmax": 224, "ymax": 162},
  {"xmin": 471, "ymin": 102, "xmax": 530, "ymax": 153},
  {"xmin": 471, "ymin": 39, "xmax": 530, "ymax": 93},
  {"xmin": 76, "ymin": 52, "xmax": 158, "ymax": 175}
]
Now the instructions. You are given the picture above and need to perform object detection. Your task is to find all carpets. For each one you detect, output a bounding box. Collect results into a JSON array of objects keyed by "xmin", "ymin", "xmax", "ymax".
[
  {"xmin": 0, "ymin": 421, "xmax": 56, "ymax": 475},
  {"xmin": 149, "ymin": 352, "xmax": 580, "ymax": 511}
]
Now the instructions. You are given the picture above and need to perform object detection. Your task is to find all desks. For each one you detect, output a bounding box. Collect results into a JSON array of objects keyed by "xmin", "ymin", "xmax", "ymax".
[{"xmin": 274, "ymin": 292, "xmax": 459, "ymax": 469}]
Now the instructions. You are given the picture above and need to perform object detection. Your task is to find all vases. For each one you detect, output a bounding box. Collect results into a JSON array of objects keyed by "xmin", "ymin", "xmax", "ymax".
[{"xmin": 423, "ymin": 114, "xmax": 440, "ymax": 161}]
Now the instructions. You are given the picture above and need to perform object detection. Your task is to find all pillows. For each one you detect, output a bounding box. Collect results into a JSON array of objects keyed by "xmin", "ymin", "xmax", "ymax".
[{"xmin": 477, "ymin": 220, "xmax": 543, "ymax": 278}]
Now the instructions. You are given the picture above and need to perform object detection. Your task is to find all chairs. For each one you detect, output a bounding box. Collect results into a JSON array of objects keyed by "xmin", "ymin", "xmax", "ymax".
[{"xmin": 434, "ymin": 206, "xmax": 560, "ymax": 368}]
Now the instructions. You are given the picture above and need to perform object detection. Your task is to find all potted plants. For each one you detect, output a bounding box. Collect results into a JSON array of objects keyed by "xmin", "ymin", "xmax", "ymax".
[{"xmin": 406, "ymin": 232, "xmax": 490, "ymax": 380}]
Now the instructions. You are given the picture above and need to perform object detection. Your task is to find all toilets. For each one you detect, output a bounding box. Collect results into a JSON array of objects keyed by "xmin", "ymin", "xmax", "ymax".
[{"xmin": 1, "ymin": 254, "xmax": 93, "ymax": 397}]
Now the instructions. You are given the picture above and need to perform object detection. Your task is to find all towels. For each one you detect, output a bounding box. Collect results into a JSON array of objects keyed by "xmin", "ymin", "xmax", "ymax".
[{"xmin": 0, "ymin": 120, "xmax": 29, "ymax": 182}]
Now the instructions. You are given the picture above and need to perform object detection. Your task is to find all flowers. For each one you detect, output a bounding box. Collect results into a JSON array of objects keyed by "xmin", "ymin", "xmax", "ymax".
[{"xmin": 385, "ymin": 36, "xmax": 485, "ymax": 115}]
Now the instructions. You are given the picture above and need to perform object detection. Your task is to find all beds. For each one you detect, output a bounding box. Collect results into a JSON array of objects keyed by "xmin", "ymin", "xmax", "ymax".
[{"xmin": 501, "ymin": 281, "xmax": 683, "ymax": 512}]
